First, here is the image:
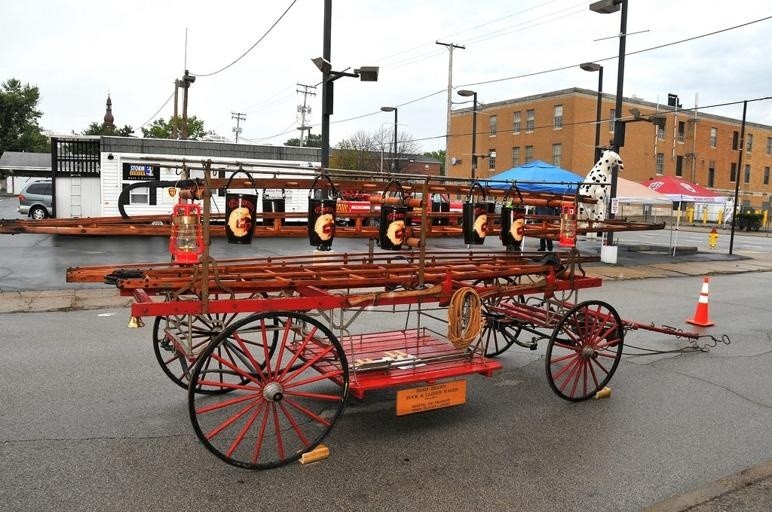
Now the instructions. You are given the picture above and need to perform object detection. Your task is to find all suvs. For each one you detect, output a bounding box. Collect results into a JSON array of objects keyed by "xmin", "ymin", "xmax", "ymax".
[{"xmin": 17, "ymin": 177, "xmax": 54, "ymax": 220}]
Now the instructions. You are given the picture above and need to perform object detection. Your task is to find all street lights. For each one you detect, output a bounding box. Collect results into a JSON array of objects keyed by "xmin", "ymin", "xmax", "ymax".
[
  {"xmin": 458, "ymin": 90, "xmax": 477, "ymax": 179},
  {"xmin": 587, "ymin": 0, "xmax": 631, "ymax": 245},
  {"xmin": 579, "ymin": 62, "xmax": 604, "ymax": 166},
  {"xmin": 381, "ymin": 106, "xmax": 399, "ymax": 172}
]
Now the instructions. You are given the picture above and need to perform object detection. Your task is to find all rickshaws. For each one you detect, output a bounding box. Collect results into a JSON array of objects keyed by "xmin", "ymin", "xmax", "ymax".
[{"xmin": 65, "ymin": 245, "xmax": 732, "ymax": 472}]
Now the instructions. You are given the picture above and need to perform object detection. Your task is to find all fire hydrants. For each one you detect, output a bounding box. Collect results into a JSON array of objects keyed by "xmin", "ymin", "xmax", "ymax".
[{"xmin": 708, "ymin": 228, "xmax": 719, "ymax": 249}]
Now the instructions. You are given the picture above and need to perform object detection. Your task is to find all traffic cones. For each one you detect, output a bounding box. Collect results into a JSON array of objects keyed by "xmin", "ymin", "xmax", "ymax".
[{"xmin": 686, "ymin": 277, "xmax": 715, "ymax": 328}]
